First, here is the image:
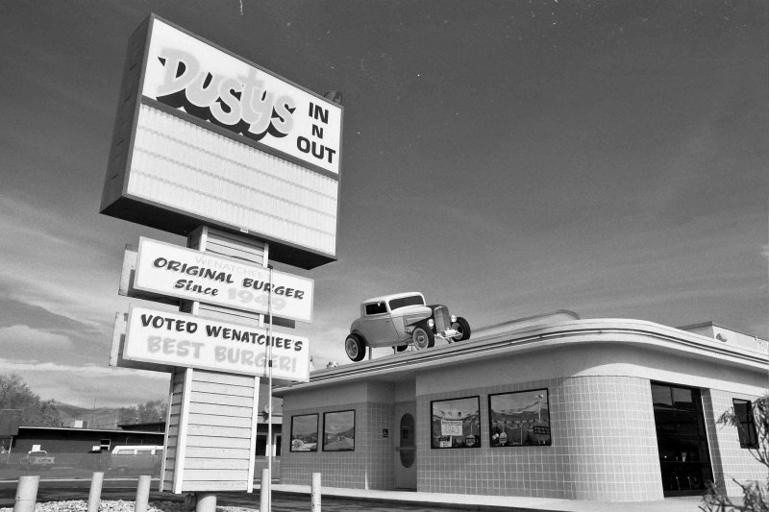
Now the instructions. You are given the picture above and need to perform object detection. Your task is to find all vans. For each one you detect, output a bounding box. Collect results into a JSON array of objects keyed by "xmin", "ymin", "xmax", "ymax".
[{"xmin": 110, "ymin": 446, "xmax": 164, "ymax": 474}]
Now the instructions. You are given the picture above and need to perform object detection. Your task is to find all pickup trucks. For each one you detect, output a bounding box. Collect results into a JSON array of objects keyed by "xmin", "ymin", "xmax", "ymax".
[{"xmin": 20, "ymin": 451, "xmax": 55, "ymax": 471}]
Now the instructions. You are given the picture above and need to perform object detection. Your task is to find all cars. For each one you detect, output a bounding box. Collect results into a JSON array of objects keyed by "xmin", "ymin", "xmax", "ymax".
[{"xmin": 343, "ymin": 291, "xmax": 473, "ymax": 363}]
